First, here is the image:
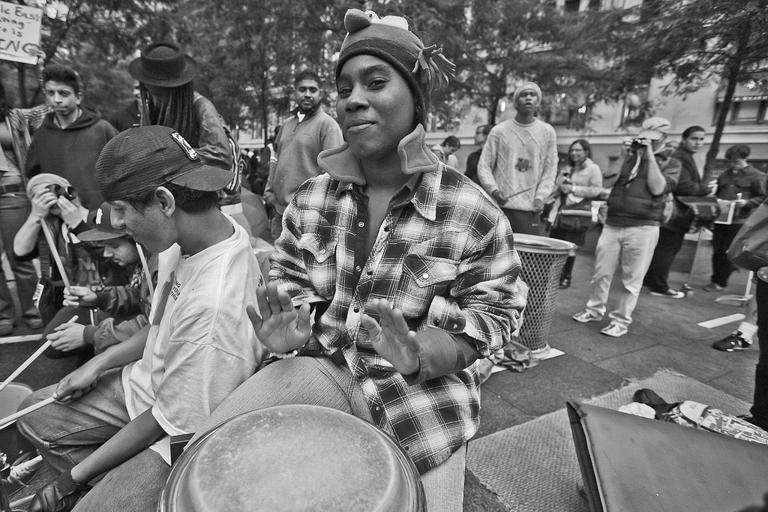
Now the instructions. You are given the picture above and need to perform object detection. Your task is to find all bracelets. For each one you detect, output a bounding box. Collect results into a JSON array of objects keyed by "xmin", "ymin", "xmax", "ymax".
[{"xmin": 268, "ymin": 343, "xmax": 307, "ymax": 359}]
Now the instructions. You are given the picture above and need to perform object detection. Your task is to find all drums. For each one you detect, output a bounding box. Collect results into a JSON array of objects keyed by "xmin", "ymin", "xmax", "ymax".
[
  {"xmin": 0, "ymin": 383, "xmax": 35, "ymax": 477},
  {"xmin": 513, "ymin": 231, "xmax": 578, "ymax": 356},
  {"xmin": 158, "ymin": 404, "xmax": 429, "ymax": 512}
]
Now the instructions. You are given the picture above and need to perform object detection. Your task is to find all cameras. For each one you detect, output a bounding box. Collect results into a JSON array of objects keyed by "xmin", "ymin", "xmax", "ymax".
[
  {"xmin": 629, "ymin": 137, "xmax": 646, "ymax": 149},
  {"xmin": 47, "ymin": 184, "xmax": 76, "ymax": 210}
]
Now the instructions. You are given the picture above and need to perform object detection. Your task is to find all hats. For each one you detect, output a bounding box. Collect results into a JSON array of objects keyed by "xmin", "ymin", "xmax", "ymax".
[
  {"xmin": 337, "ymin": 8, "xmax": 456, "ymax": 128},
  {"xmin": 512, "ymin": 81, "xmax": 543, "ymax": 108},
  {"xmin": 128, "ymin": 43, "xmax": 199, "ymax": 87},
  {"xmin": 636, "ymin": 117, "xmax": 670, "ymax": 140},
  {"xmin": 96, "ymin": 125, "xmax": 235, "ymax": 203},
  {"xmin": 76, "ymin": 201, "xmax": 126, "ymax": 242}
]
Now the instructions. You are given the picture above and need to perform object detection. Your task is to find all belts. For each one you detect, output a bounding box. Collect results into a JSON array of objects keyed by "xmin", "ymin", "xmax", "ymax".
[{"xmin": 0, "ymin": 184, "xmax": 21, "ymax": 193}]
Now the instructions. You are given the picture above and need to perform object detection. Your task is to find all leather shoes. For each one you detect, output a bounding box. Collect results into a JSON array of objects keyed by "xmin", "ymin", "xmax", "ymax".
[{"xmin": 560, "ymin": 274, "xmax": 571, "ymax": 289}]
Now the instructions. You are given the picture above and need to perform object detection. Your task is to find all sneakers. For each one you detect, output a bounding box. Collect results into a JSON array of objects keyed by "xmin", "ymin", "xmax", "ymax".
[
  {"xmin": 650, "ymin": 289, "xmax": 685, "ymax": 299},
  {"xmin": 599, "ymin": 320, "xmax": 629, "ymax": 337},
  {"xmin": 24, "ymin": 312, "xmax": 44, "ymax": 329},
  {"xmin": 703, "ymin": 283, "xmax": 724, "ymax": 292},
  {"xmin": 713, "ymin": 331, "xmax": 752, "ymax": 350},
  {"xmin": 572, "ymin": 310, "xmax": 602, "ymax": 322},
  {"xmin": 0, "ymin": 317, "xmax": 15, "ymax": 336}
]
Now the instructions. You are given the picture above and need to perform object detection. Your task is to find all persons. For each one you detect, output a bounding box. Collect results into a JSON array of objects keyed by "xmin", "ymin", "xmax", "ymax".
[
  {"xmin": 241, "ymin": 69, "xmax": 343, "ymax": 241},
  {"xmin": 179, "ymin": 9, "xmax": 529, "ymax": 512},
  {"xmin": 431, "ymin": 80, "xmax": 557, "ymax": 234},
  {"xmin": 573, "ymin": 118, "xmax": 767, "ymax": 430},
  {"xmin": 546, "ymin": 141, "xmax": 603, "ymax": 289},
  {"xmin": 16, "ymin": 123, "xmax": 267, "ymax": 512},
  {"xmin": 0, "ymin": 43, "xmax": 242, "ymax": 357}
]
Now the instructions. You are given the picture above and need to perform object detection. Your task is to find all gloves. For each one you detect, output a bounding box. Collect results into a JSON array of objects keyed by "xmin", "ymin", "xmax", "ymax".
[{"xmin": 28, "ymin": 471, "xmax": 84, "ymax": 512}]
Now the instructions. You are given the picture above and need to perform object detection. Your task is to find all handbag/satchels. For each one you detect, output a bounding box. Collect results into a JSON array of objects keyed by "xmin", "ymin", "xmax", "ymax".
[
  {"xmin": 726, "ymin": 198, "xmax": 768, "ymax": 269},
  {"xmin": 557, "ymin": 199, "xmax": 592, "ymax": 234}
]
